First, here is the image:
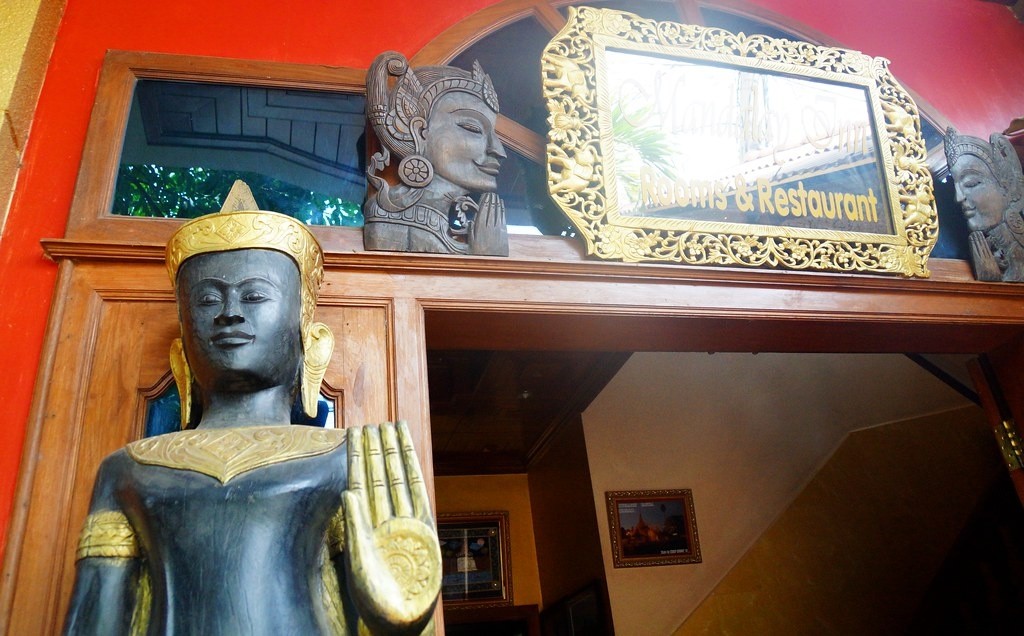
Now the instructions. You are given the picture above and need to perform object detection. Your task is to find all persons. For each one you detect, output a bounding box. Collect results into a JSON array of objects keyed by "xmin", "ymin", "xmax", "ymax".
[
  {"xmin": 60, "ymin": 181, "xmax": 443, "ymax": 636},
  {"xmin": 360, "ymin": 52, "xmax": 510, "ymax": 257},
  {"xmin": 943, "ymin": 127, "xmax": 1024, "ymax": 283}
]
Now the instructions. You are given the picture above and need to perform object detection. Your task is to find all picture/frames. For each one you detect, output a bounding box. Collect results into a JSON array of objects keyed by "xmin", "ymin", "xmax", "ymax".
[
  {"xmin": 565, "ymin": 584, "xmax": 603, "ymax": 636},
  {"xmin": 434, "ymin": 510, "xmax": 514, "ymax": 611},
  {"xmin": 605, "ymin": 488, "xmax": 703, "ymax": 569}
]
